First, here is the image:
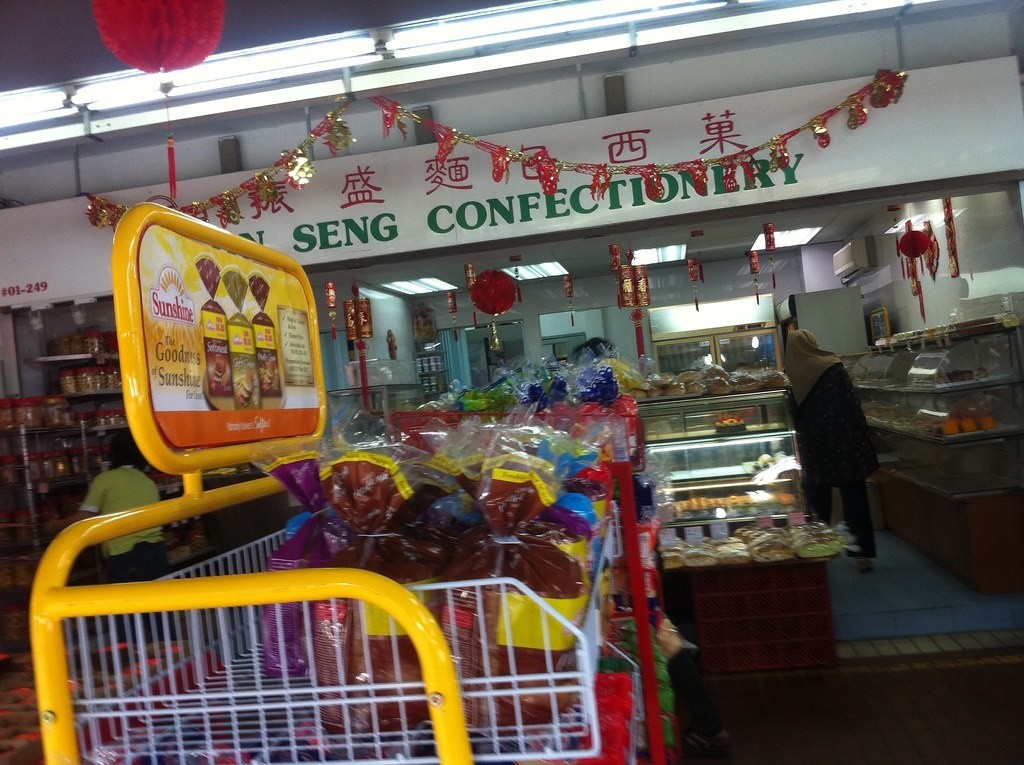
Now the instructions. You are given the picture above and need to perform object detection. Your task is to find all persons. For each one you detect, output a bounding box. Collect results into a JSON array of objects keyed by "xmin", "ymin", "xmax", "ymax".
[
  {"xmin": 785, "ymin": 329, "xmax": 880, "ymax": 572},
  {"xmin": 42, "ymin": 427, "xmax": 171, "ymax": 643}
]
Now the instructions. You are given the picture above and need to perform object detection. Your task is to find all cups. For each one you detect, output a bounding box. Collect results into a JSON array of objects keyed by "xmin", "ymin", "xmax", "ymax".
[{"xmin": 418, "ymin": 356, "xmax": 443, "ymax": 392}]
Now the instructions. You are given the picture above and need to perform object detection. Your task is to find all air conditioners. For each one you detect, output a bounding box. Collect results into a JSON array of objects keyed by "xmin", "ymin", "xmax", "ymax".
[{"xmin": 833, "ymin": 236, "xmax": 877, "ymax": 284}]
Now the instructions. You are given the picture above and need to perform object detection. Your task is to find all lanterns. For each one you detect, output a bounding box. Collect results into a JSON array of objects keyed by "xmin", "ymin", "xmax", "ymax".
[
  {"xmin": 899, "ymin": 230, "xmax": 928, "ymax": 323},
  {"xmin": 471, "ymin": 269, "xmax": 517, "ymax": 349},
  {"xmin": 92, "ymin": 0, "xmax": 224, "ymax": 203}
]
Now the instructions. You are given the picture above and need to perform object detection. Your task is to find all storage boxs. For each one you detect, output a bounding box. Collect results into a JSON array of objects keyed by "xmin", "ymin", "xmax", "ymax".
[{"xmin": 689, "ymin": 562, "xmax": 838, "ymax": 674}]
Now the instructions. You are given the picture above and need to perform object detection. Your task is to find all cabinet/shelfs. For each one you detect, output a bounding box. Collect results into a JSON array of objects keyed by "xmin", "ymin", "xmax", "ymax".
[
  {"xmin": 851, "ymin": 312, "xmax": 1024, "ymax": 595},
  {"xmin": 636, "ymin": 369, "xmax": 811, "ymax": 540},
  {"xmin": 0, "ymin": 329, "xmax": 259, "ymax": 650}
]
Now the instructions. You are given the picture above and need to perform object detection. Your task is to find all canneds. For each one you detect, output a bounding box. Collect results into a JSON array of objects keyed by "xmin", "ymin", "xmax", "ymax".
[
  {"xmin": 414, "ymin": 355, "xmax": 444, "ymax": 392},
  {"xmin": 0, "ymin": 330, "xmax": 209, "ymax": 644}
]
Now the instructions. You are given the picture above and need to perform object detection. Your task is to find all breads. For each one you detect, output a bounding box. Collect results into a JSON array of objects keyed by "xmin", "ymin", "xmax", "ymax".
[
  {"xmin": 934, "ymin": 370, "xmax": 974, "ymax": 385},
  {"xmin": 657, "ymin": 493, "xmax": 844, "ymax": 570},
  {"xmin": 624, "ymin": 366, "xmax": 784, "ymax": 400},
  {"xmin": 921, "ymin": 411, "xmax": 996, "ymax": 435}
]
